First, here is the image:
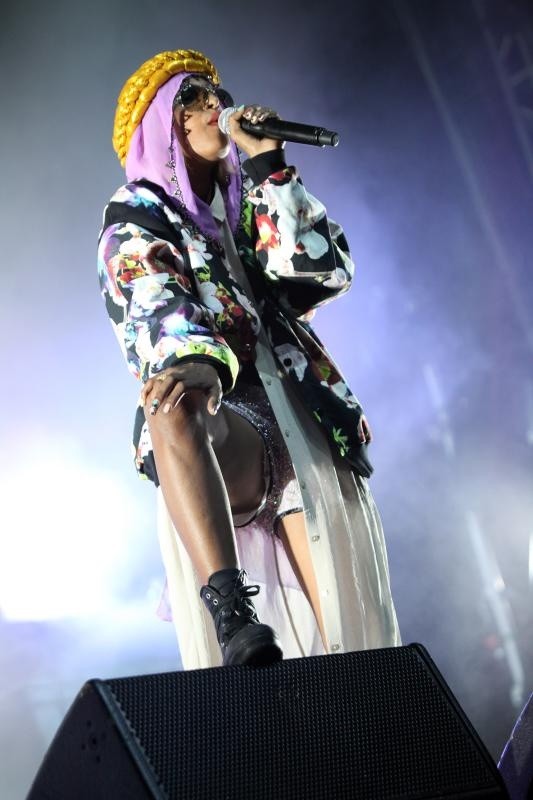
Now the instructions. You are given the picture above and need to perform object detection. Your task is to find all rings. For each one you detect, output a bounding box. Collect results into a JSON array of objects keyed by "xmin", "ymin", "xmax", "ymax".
[{"xmin": 156, "ymin": 372, "xmax": 167, "ymax": 381}]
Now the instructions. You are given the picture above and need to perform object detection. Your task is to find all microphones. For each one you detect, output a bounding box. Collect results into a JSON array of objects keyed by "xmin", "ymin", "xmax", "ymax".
[{"xmin": 218, "ymin": 107, "xmax": 340, "ymax": 148}]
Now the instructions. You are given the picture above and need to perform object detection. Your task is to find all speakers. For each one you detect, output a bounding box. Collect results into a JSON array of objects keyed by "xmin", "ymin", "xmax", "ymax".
[
  {"xmin": 23, "ymin": 640, "xmax": 512, "ymax": 800},
  {"xmin": 497, "ymin": 692, "xmax": 533, "ymax": 800}
]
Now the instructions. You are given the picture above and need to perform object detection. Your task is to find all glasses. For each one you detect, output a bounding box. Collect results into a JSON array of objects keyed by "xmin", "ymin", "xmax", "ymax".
[{"xmin": 172, "ymin": 83, "xmax": 236, "ymax": 112}]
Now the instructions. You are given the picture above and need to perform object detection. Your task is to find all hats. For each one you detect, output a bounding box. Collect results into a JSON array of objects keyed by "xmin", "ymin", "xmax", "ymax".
[{"xmin": 112, "ymin": 48, "xmax": 222, "ymax": 167}]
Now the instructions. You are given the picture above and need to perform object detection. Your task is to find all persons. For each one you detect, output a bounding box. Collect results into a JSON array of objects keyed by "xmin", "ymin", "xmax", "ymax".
[{"xmin": 95, "ymin": 49, "xmax": 403, "ymax": 669}]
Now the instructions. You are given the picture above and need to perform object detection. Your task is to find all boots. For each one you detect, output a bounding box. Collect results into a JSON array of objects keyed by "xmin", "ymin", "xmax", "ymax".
[{"xmin": 199, "ymin": 566, "xmax": 286, "ymax": 667}]
